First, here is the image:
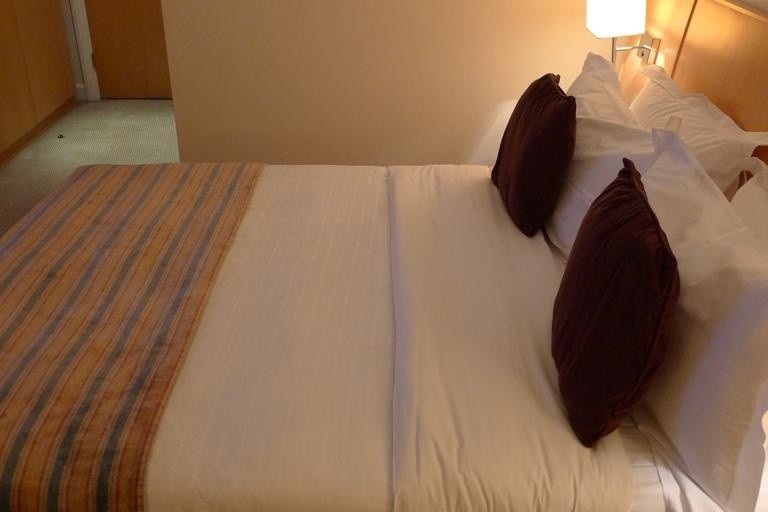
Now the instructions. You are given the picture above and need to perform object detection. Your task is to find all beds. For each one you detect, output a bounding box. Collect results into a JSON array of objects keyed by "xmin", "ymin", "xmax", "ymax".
[{"xmin": 0, "ymin": 0, "xmax": 768, "ymax": 512}]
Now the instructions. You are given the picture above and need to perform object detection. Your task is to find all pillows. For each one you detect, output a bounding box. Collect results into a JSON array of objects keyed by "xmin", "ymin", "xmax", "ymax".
[
  {"xmin": 627, "ymin": 64, "xmax": 767, "ymax": 194},
  {"xmin": 490, "ymin": 73, "xmax": 578, "ymax": 238},
  {"xmin": 730, "ymin": 156, "xmax": 767, "ymax": 247},
  {"xmin": 545, "ymin": 51, "xmax": 660, "ymax": 261},
  {"xmin": 551, "ymin": 156, "xmax": 683, "ymax": 448},
  {"xmin": 641, "ymin": 127, "xmax": 768, "ymax": 511}
]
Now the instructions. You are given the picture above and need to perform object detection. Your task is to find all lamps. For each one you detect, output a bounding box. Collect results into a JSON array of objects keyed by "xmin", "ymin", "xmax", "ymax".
[{"xmin": 585, "ymin": 0, "xmax": 646, "ymax": 66}]
